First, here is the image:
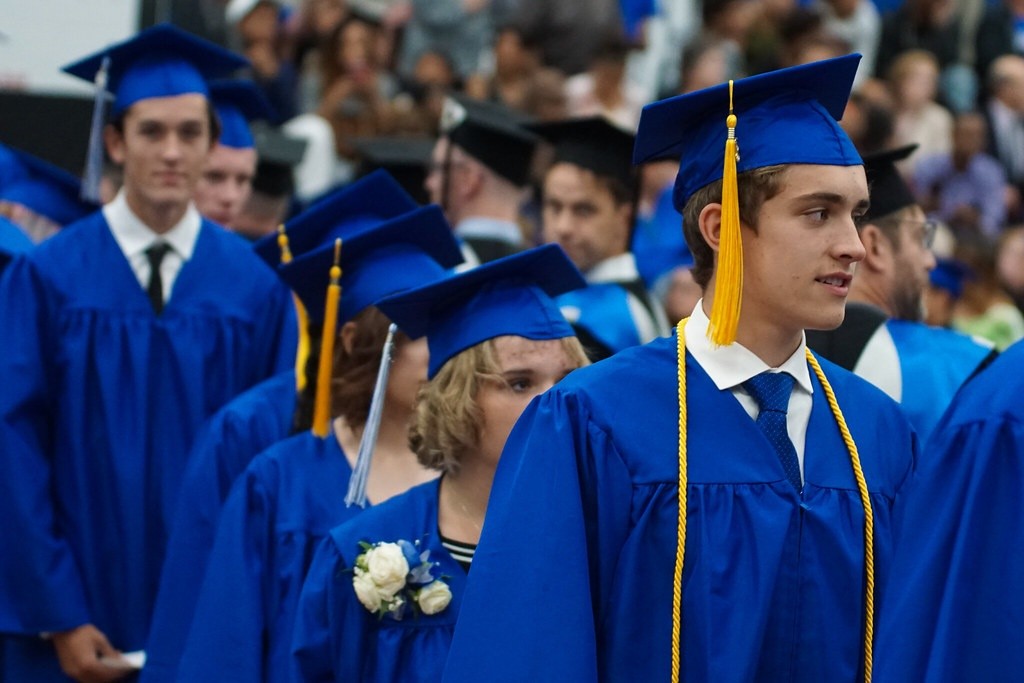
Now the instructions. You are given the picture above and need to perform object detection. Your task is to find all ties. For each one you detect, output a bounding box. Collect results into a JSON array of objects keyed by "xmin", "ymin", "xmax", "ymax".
[
  {"xmin": 742, "ymin": 372, "xmax": 802, "ymax": 494},
  {"xmin": 145, "ymin": 243, "xmax": 172, "ymax": 315}
]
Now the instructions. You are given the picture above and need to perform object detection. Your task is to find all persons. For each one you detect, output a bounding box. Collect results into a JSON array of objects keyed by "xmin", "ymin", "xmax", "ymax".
[
  {"xmin": 444, "ymin": 54, "xmax": 923, "ymax": 683},
  {"xmin": 0, "ymin": 0, "xmax": 1024, "ymax": 682}
]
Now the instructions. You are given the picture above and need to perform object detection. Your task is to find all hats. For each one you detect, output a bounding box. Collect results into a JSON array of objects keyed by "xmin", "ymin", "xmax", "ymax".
[
  {"xmin": 252, "ymin": 168, "xmax": 417, "ymax": 391},
  {"xmin": 347, "ymin": 135, "xmax": 432, "ymax": 192},
  {"xmin": 64, "ymin": 21, "xmax": 250, "ymax": 201},
  {"xmin": 344, "ymin": 244, "xmax": 587, "ymax": 507},
  {"xmin": 855, "ymin": 144, "xmax": 918, "ymax": 223},
  {"xmin": 631, "ymin": 52, "xmax": 865, "ymax": 351},
  {"xmin": 0, "ymin": 215, "xmax": 34, "ymax": 258},
  {"xmin": 254, "ymin": 158, "xmax": 293, "ymax": 198},
  {"xmin": 277, "ymin": 204, "xmax": 465, "ymax": 438},
  {"xmin": 208, "ymin": 78, "xmax": 254, "ymax": 148},
  {"xmin": 929, "ymin": 258, "xmax": 978, "ymax": 298},
  {"xmin": 439, "ymin": 96, "xmax": 538, "ymax": 210},
  {"xmin": 519, "ymin": 114, "xmax": 641, "ymax": 198}
]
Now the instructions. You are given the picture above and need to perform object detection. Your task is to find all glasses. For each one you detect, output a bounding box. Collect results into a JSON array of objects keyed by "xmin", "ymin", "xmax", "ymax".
[{"xmin": 887, "ymin": 220, "xmax": 937, "ymax": 249}]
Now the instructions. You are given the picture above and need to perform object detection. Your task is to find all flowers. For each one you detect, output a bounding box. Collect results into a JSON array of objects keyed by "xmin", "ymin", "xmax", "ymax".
[{"xmin": 352, "ymin": 539, "xmax": 452, "ymax": 620}]
